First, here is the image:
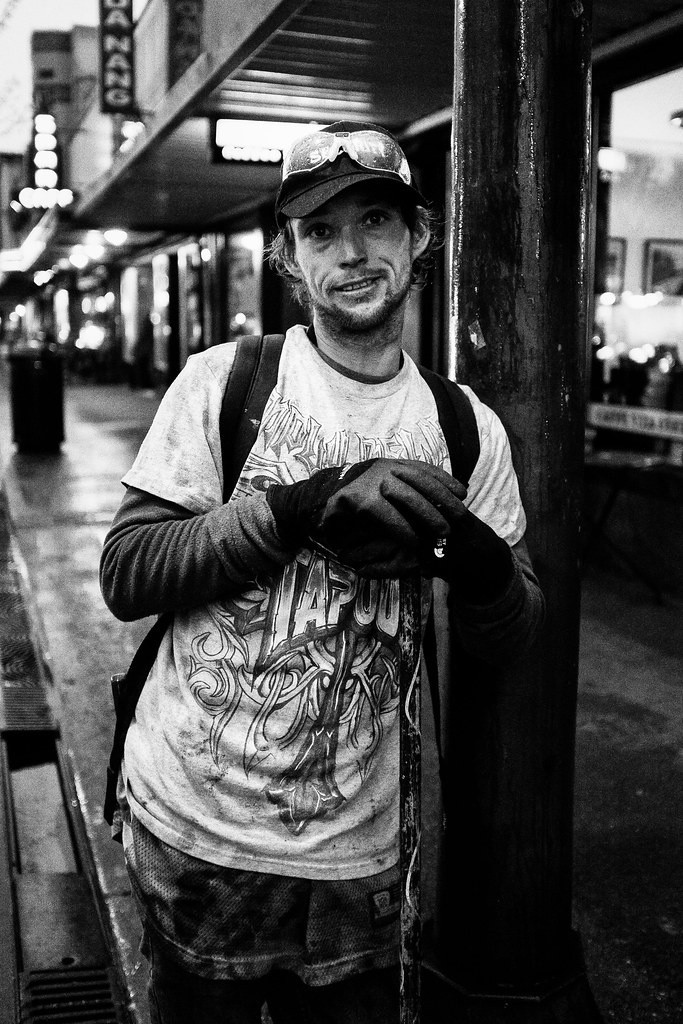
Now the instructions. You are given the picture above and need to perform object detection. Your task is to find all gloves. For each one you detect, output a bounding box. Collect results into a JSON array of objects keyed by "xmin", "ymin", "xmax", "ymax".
[
  {"xmin": 268, "ymin": 458, "xmax": 469, "ymax": 559},
  {"xmin": 354, "ymin": 508, "xmax": 514, "ymax": 602}
]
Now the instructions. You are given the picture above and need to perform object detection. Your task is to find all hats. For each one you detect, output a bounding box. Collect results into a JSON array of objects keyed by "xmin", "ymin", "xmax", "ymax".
[{"xmin": 275, "ymin": 121, "xmax": 429, "ymax": 231}]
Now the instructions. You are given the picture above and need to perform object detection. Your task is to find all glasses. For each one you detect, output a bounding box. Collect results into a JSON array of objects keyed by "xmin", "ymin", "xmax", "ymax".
[{"xmin": 282, "ymin": 131, "xmax": 411, "ymax": 185}]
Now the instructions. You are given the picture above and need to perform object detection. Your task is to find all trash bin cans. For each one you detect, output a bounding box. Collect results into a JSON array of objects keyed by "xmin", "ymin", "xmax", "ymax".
[{"xmin": 5, "ymin": 349, "xmax": 67, "ymax": 455}]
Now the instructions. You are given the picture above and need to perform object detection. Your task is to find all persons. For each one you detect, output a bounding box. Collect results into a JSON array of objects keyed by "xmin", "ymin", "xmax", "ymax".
[{"xmin": 97, "ymin": 122, "xmax": 547, "ymax": 1024}]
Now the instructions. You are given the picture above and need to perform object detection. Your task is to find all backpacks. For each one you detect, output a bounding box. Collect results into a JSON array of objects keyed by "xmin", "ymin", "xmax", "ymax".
[{"xmin": 103, "ymin": 334, "xmax": 483, "ymax": 825}]
